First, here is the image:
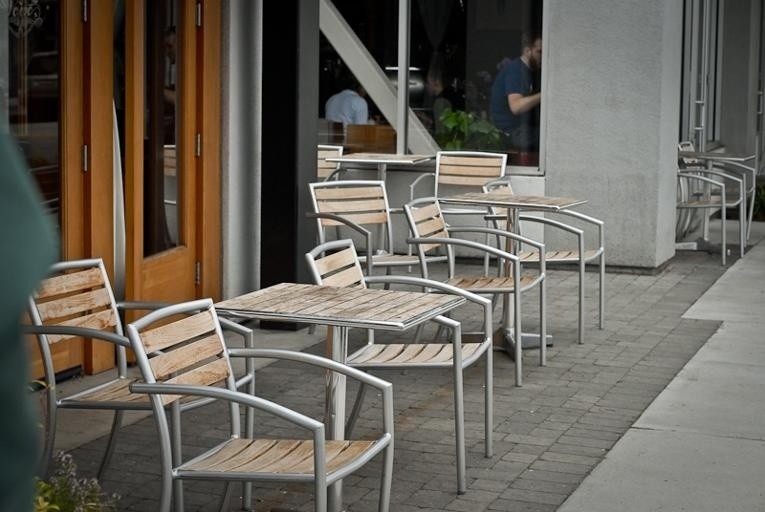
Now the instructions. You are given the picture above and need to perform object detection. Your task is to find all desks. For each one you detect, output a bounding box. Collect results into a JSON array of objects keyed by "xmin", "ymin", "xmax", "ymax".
[{"xmin": 678, "ymin": 152, "xmax": 754, "ymax": 254}]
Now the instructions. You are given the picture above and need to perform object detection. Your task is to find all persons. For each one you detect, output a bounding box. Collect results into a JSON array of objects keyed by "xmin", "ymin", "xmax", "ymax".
[
  {"xmin": 426, "ymin": 69, "xmax": 465, "ymax": 135},
  {"xmin": 325, "ymin": 81, "xmax": 380, "ymax": 126},
  {"xmin": 163, "ymin": 25, "xmax": 176, "ymax": 107},
  {"xmin": 488, "ymin": 33, "xmax": 541, "ymax": 152}
]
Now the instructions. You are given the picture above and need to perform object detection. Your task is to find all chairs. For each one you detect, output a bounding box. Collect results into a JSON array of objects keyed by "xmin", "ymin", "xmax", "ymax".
[
  {"xmin": 318, "ymin": 120, "xmax": 344, "ymax": 145},
  {"xmin": 678, "ymin": 141, "xmax": 755, "ymax": 246},
  {"xmin": 677, "ymin": 145, "xmax": 743, "ymax": 265}
]
